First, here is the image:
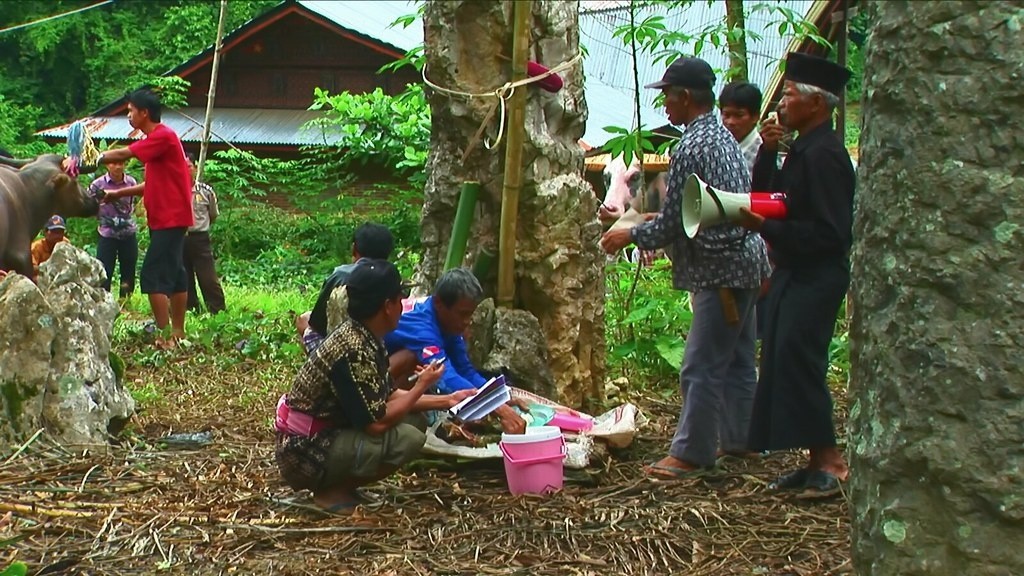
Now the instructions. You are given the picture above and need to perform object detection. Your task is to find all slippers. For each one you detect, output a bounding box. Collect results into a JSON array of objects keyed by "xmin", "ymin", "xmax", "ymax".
[
  {"xmin": 303, "ymin": 500, "xmax": 363, "ymax": 521},
  {"xmin": 714, "ymin": 446, "xmax": 761, "ymax": 462},
  {"xmin": 766, "ymin": 467, "xmax": 849, "ymax": 500},
  {"xmin": 639, "ymin": 460, "xmax": 707, "ymax": 484}
]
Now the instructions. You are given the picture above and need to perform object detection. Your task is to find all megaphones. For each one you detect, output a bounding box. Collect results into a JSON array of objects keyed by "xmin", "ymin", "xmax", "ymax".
[{"xmin": 681, "ymin": 173, "xmax": 789, "ymax": 239}]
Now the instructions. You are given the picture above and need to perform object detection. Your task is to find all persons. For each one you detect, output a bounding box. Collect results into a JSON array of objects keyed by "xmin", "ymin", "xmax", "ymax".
[
  {"xmin": 274, "ymin": 221, "xmax": 531, "ymax": 517},
  {"xmin": 599, "ymin": 52, "xmax": 857, "ymax": 499},
  {"xmin": 63, "ymin": 89, "xmax": 226, "ymax": 339},
  {"xmin": 31, "ymin": 215, "xmax": 70, "ymax": 272}
]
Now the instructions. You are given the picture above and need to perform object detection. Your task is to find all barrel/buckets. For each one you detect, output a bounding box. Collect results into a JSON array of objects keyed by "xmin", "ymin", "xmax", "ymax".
[{"xmin": 498, "ymin": 426, "xmax": 569, "ymax": 497}]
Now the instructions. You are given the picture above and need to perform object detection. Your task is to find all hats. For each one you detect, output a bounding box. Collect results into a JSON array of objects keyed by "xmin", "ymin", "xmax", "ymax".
[
  {"xmin": 785, "ymin": 51, "xmax": 852, "ymax": 99},
  {"xmin": 42, "ymin": 215, "xmax": 65, "ymax": 229},
  {"xmin": 644, "ymin": 57, "xmax": 716, "ymax": 88},
  {"xmin": 345, "ymin": 262, "xmax": 420, "ymax": 300}
]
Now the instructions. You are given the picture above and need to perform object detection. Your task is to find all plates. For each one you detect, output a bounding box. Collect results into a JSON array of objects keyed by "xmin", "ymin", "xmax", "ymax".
[{"xmin": 497, "ymin": 401, "xmax": 555, "ymax": 426}]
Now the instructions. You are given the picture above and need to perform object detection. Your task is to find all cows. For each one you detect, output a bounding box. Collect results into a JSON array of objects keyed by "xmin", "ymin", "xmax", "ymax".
[
  {"xmin": 584, "ymin": 152, "xmax": 668, "ymax": 260},
  {"xmin": 0, "ymin": 153, "xmax": 100, "ymax": 283}
]
